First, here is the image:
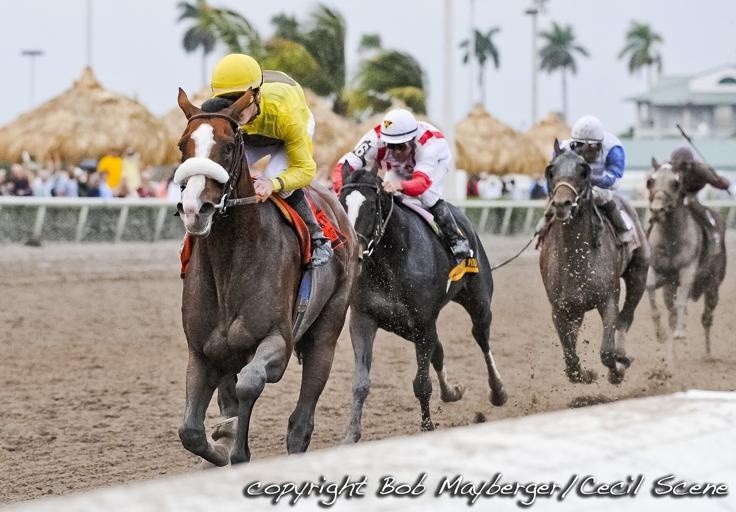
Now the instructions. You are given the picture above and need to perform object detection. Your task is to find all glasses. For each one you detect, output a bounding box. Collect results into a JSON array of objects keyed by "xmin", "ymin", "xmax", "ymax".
[{"xmin": 387, "ymin": 143, "xmax": 405, "ymax": 150}]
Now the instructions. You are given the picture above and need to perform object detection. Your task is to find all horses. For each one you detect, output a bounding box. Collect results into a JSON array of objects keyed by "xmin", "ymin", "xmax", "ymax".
[
  {"xmin": 171, "ymin": 85, "xmax": 360, "ymax": 467},
  {"xmin": 337, "ymin": 156, "xmax": 509, "ymax": 445},
  {"xmin": 644, "ymin": 154, "xmax": 730, "ymax": 364},
  {"xmin": 536, "ymin": 136, "xmax": 650, "ymax": 387}
]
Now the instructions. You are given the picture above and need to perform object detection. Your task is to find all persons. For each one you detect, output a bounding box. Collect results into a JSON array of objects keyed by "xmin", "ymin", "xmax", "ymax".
[
  {"xmin": 210, "ymin": 51, "xmax": 337, "ymax": 267},
  {"xmin": 669, "ymin": 147, "xmax": 729, "ymax": 302},
  {"xmin": 0, "ymin": 150, "xmax": 549, "ymax": 199},
  {"xmin": 548, "ymin": 117, "xmax": 633, "ymax": 245},
  {"xmin": 330, "ymin": 108, "xmax": 479, "ymax": 278}
]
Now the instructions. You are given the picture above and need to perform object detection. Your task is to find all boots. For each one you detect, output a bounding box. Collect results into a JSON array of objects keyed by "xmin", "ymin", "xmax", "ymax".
[
  {"xmin": 429, "ymin": 199, "xmax": 467, "ymax": 256},
  {"xmin": 600, "ymin": 200, "xmax": 634, "ymax": 242},
  {"xmin": 283, "ymin": 189, "xmax": 331, "ymax": 267}
]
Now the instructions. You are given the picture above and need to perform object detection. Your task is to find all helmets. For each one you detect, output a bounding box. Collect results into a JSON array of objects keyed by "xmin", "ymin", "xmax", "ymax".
[
  {"xmin": 379, "ymin": 110, "xmax": 418, "ymax": 144},
  {"xmin": 671, "ymin": 146, "xmax": 693, "ymax": 164},
  {"xmin": 570, "ymin": 117, "xmax": 604, "ymax": 144},
  {"xmin": 211, "ymin": 54, "xmax": 263, "ymax": 97}
]
